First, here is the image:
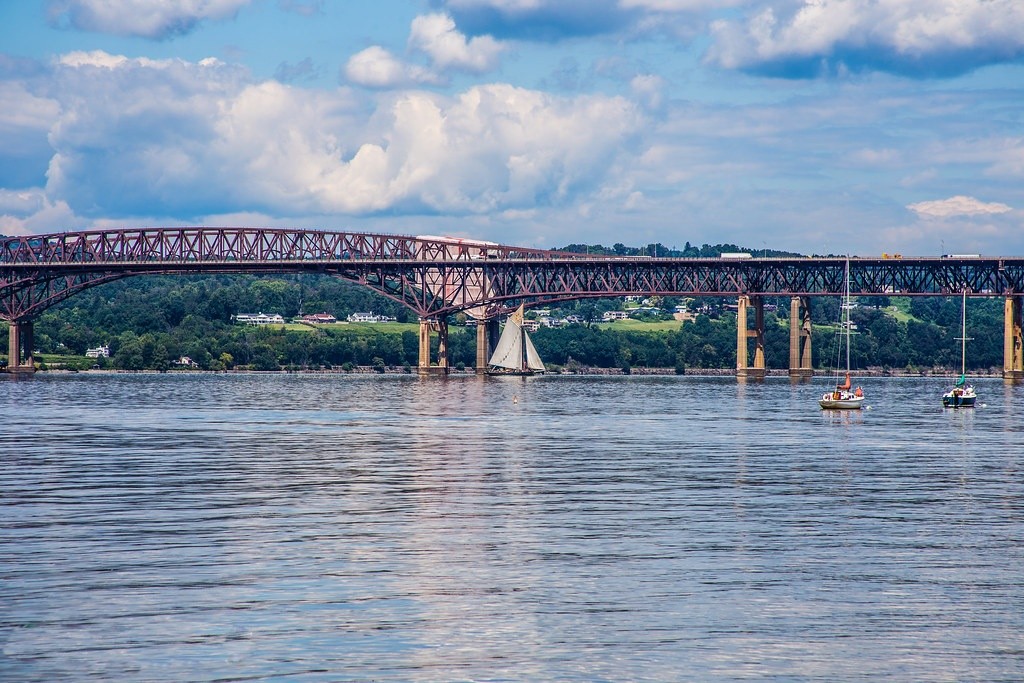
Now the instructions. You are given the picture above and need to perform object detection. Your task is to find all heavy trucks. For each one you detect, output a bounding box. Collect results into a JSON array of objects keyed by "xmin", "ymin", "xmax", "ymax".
[
  {"xmin": 453, "ymin": 255, "xmax": 480, "ymax": 259},
  {"xmin": 721, "ymin": 253, "xmax": 752, "ymax": 259},
  {"xmin": 941, "ymin": 254, "xmax": 981, "ymax": 258}
]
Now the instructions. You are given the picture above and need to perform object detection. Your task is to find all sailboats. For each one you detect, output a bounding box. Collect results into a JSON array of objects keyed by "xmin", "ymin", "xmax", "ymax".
[
  {"xmin": 819, "ymin": 254, "xmax": 865, "ymax": 410},
  {"xmin": 485, "ymin": 303, "xmax": 547, "ymax": 376},
  {"xmin": 942, "ymin": 291, "xmax": 977, "ymax": 408}
]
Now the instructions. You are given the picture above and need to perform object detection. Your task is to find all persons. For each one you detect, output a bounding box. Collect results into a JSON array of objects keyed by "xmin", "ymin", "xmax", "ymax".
[
  {"xmin": 966, "ymin": 384, "xmax": 973, "ymax": 394},
  {"xmin": 856, "ymin": 386, "xmax": 862, "ymax": 397}
]
